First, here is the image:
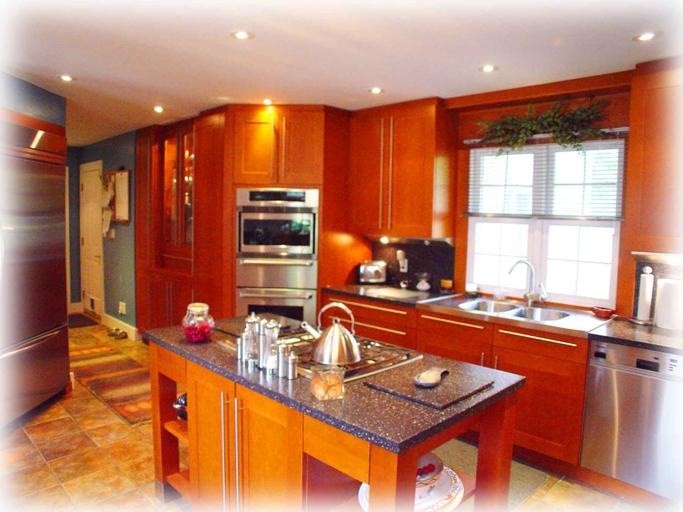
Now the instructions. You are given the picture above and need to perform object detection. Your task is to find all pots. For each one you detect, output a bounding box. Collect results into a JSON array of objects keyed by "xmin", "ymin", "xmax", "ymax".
[{"xmin": 359, "ymin": 260, "xmax": 387, "ymax": 283}]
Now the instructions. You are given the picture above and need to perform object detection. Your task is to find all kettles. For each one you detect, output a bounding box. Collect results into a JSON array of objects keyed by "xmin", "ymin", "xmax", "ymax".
[{"xmin": 300, "ymin": 300, "xmax": 361, "ymax": 366}]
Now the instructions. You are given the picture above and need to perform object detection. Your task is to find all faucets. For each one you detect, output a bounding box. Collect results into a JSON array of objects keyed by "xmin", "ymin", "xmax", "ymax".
[{"xmin": 508, "ymin": 259, "xmax": 544, "ymax": 308}]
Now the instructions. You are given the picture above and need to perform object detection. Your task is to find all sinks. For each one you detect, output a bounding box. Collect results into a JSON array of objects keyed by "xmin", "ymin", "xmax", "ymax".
[
  {"xmin": 472, "ymin": 297, "xmax": 518, "ymax": 314},
  {"xmin": 514, "ymin": 306, "xmax": 571, "ymax": 324}
]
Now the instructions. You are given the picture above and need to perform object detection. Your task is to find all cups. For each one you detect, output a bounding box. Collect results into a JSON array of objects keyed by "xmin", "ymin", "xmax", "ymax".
[{"xmin": 312, "ymin": 368, "xmax": 346, "ymax": 401}]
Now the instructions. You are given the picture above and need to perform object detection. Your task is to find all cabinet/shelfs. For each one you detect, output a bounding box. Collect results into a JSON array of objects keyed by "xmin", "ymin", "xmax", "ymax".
[
  {"xmin": 415, "ymin": 310, "xmax": 587, "ymax": 484},
  {"xmin": 185, "ymin": 355, "xmax": 304, "ymax": 512},
  {"xmin": 322, "ymin": 295, "xmax": 413, "ymax": 350},
  {"xmin": 147, "ymin": 271, "xmax": 192, "ymax": 332},
  {"xmin": 234, "ymin": 104, "xmax": 324, "ymax": 187},
  {"xmin": 345, "ymin": 98, "xmax": 433, "ymax": 238},
  {"xmin": 140, "ymin": 311, "xmax": 527, "ymax": 512},
  {"xmin": 147, "ymin": 122, "xmax": 196, "ymax": 280}
]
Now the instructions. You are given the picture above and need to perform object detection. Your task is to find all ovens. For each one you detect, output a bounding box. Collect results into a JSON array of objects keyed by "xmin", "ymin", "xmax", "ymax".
[
  {"xmin": 236, "ymin": 289, "xmax": 316, "ymax": 328},
  {"xmin": 236, "ymin": 188, "xmax": 316, "ymax": 288}
]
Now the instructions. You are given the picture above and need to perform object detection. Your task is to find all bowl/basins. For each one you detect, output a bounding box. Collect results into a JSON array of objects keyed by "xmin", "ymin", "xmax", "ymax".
[{"xmin": 593, "ymin": 307, "xmax": 613, "ymax": 318}]
